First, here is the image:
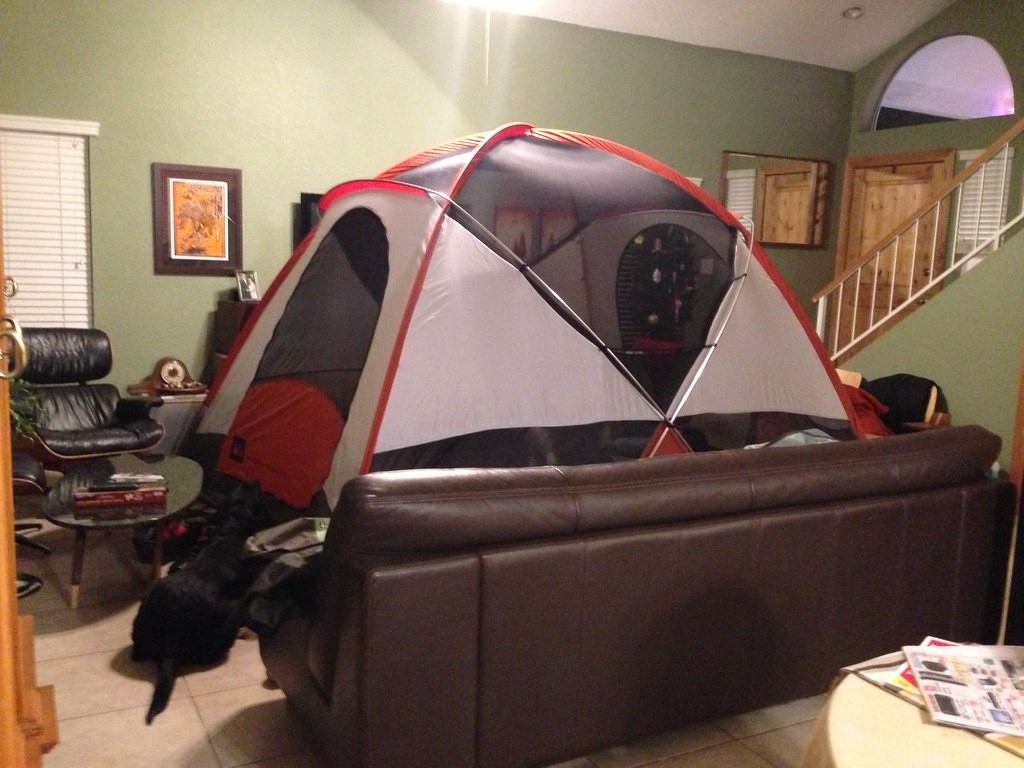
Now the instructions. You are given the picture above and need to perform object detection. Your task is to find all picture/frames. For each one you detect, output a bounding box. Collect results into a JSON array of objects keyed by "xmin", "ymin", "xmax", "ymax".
[{"xmin": 152, "ymin": 162, "xmax": 244, "ymax": 276}]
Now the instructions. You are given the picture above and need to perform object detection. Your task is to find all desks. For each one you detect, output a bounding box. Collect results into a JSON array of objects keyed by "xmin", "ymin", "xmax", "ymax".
[
  {"xmin": 796, "ymin": 649, "xmax": 1023, "ymax": 768},
  {"xmin": 42, "ymin": 453, "xmax": 203, "ymax": 585}
]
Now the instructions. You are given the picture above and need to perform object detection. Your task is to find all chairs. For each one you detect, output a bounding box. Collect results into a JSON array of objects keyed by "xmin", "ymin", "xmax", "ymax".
[
  {"xmin": 6, "ymin": 327, "xmax": 165, "ymax": 557},
  {"xmin": 836, "ymin": 369, "xmax": 938, "ymax": 422}
]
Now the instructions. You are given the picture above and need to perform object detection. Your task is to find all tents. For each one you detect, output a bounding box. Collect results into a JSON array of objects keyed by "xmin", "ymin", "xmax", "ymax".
[{"xmin": 175, "ymin": 119, "xmax": 864, "ymax": 522}]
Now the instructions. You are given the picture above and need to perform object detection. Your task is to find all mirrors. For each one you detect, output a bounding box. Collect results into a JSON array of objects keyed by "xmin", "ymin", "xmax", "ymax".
[{"xmin": 719, "ymin": 151, "xmax": 833, "ymax": 250}]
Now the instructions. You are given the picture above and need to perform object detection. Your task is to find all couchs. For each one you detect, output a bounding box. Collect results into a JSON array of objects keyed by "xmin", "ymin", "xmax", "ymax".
[{"xmin": 244, "ymin": 424, "xmax": 1017, "ymax": 768}]
